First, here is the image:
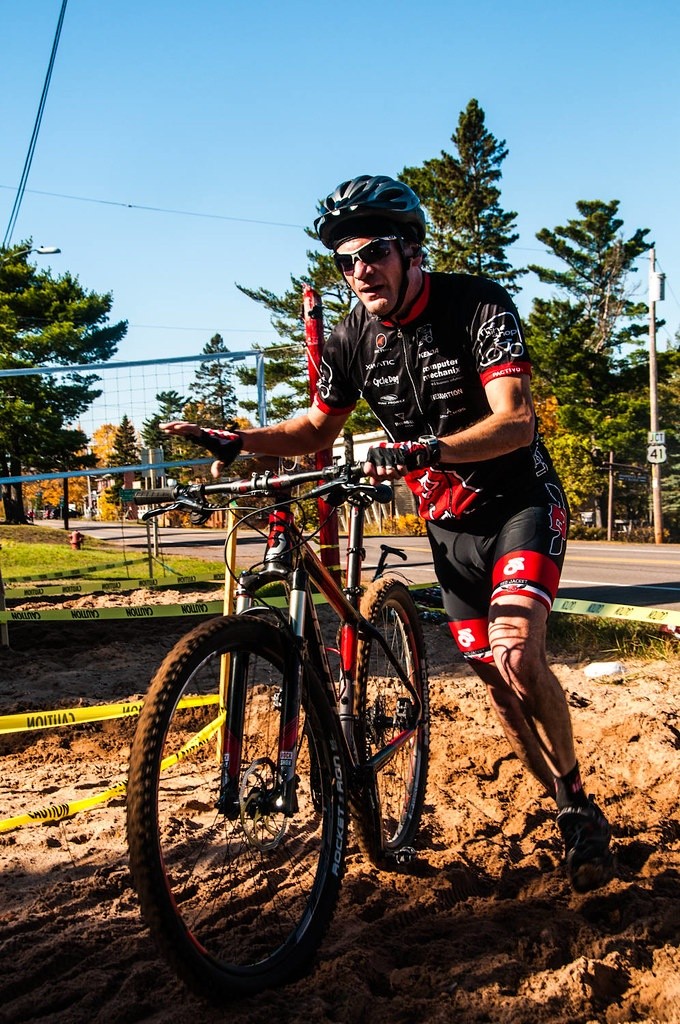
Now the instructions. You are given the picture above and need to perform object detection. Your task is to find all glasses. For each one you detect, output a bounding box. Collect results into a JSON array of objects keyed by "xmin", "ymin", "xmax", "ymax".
[{"xmin": 334, "ymin": 235, "xmax": 418, "ymax": 272}]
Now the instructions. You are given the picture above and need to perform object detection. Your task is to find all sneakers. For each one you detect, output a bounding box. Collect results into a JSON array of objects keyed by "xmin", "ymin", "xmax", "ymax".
[{"xmin": 555, "ymin": 794, "xmax": 613, "ymax": 893}]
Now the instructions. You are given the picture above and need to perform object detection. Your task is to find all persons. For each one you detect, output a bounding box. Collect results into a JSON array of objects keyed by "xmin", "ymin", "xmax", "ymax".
[
  {"xmin": 160, "ymin": 175, "xmax": 613, "ymax": 895},
  {"xmin": 29, "ymin": 509, "xmax": 35, "ymax": 522},
  {"xmin": 52, "ymin": 504, "xmax": 61, "ymax": 520},
  {"xmin": 68, "ymin": 529, "xmax": 85, "ymax": 550}
]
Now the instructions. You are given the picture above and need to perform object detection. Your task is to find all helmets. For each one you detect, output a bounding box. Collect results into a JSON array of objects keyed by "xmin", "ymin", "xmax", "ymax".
[{"xmin": 316, "ymin": 175, "xmax": 426, "ymax": 250}]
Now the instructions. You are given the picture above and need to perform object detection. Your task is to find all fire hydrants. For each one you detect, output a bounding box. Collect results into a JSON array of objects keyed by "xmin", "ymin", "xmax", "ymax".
[{"xmin": 68, "ymin": 528, "xmax": 86, "ymax": 552}]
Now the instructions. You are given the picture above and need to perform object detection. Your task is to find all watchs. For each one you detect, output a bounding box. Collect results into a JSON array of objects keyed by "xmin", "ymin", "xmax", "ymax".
[{"xmin": 417, "ymin": 434, "xmax": 441, "ymax": 465}]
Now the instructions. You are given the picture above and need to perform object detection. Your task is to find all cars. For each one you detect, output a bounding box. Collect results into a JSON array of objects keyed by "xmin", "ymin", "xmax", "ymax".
[{"xmin": 47, "ymin": 506, "xmax": 82, "ymax": 519}]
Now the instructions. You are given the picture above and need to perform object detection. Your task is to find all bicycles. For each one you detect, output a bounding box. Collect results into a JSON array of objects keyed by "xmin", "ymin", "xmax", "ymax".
[{"xmin": 123, "ymin": 456, "xmax": 432, "ymax": 1005}]
